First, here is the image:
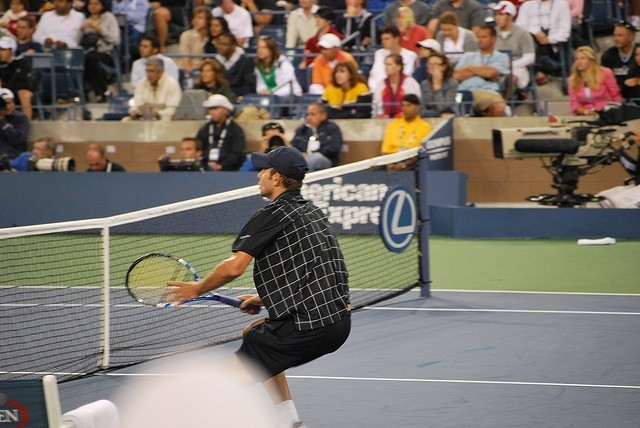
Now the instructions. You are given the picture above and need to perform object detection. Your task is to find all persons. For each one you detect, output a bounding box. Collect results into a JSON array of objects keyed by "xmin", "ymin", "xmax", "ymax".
[
  {"xmin": 419, "ymin": 51, "xmax": 458, "ymax": 116},
  {"xmin": 177, "ymin": 5, "xmax": 213, "ymax": 71},
  {"xmin": 146, "ymin": 1, "xmax": 174, "ymax": 52},
  {"xmin": 254, "ymin": 34, "xmax": 303, "ymax": 97},
  {"xmin": 0, "ymin": 0, "xmax": 36, "ymax": 37},
  {"xmin": 379, "ymin": 95, "xmax": 433, "ymax": 170},
  {"xmin": 304, "ymin": 8, "xmax": 346, "ymax": 67},
  {"xmin": 32, "ymin": 0, "xmax": 87, "ymax": 49},
  {"xmin": 213, "ymin": 30, "xmax": 253, "ymax": 97},
  {"xmin": 9, "ymin": 134, "xmax": 56, "ymax": 172},
  {"xmin": 15, "ymin": 17, "xmax": 43, "ymax": 122},
  {"xmin": 194, "ymin": 93, "xmax": 248, "ymax": 172},
  {"xmin": 450, "ymin": 27, "xmax": 511, "ymax": 117},
  {"xmin": 435, "ymin": 10, "xmax": 480, "ymax": 63},
  {"xmin": 394, "ymin": 6, "xmax": 428, "ymax": 54},
  {"xmin": 310, "ymin": 34, "xmax": 360, "ymax": 91},
  {"xmin": 367, "ymin": 28, "xmax": 417, "ymax": 89},
  {"xmin": 489, "ymin": 0, "xmax": 536, "ymax": 98},
  {"xmin": 83, "ymin": 144, "xmax": 126, "ymax": 173},
  {"xmin": 317, "ymin": 64, "xmax": 370, "ymax": 119},
  {"xmin": 0, "ymin": 36, "xmax": 27, "ymax": 88},
  {"xmin": 161, "ymin": 145, "xmax": 352, "ymax": 428},
  {"xmin": 192, "ymin": 59, "xmax": 239, "ymax": 106},
  {"xmin": 179, "ymin": 136, "xmax": 206, "ymax": 160},
  {"xmin": 291, "ymin": 104, "xmax": 343, "ymax": 173},
  {"xmin": 76, "ymin": 0, "xmax": 122, "ymax": 104},
  {"xmin": 120, "ymin": 59, "xmax": 183, "ymax": 121},
  {"xmin": 566, "ymin": 45, "xmax": 623, "ymax": 116},
  {"xmin": 130, "ymin": 38, "xmax": 180, "ymax": 88},
  {"xmin": 371, "ymin": 54, "xmax": 423, "ymax": 117},
  {"xmin": 599, "ymin": 21, "xmax": 635, "ymax": 66},
  {"xmin": 0, "ymin": 88, "xmax": 29, "ymax": 162}
]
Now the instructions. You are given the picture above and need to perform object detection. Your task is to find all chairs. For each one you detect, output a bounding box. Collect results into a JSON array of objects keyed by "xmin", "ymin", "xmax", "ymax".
[
  {"xmin": 263, "ymin": 27, "xmax": 286, "ymax": 47},
  {"xmin": 236, "ymin": 95, "xmax": 322, "ymax": 121},
  {"xmin": 106, "ymin": 94, "xmax": 133, "ymax": 121},
  {"xmin": 0, "ymin": 376, "xmax": 74, "ymax": 428},
  {"xmin": 31, "ymin": 46, "xmax": 83, "ymax": 104}
]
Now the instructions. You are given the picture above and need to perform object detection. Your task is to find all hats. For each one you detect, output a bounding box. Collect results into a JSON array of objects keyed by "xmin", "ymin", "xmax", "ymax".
[
  {"xmin": 490, "ymin": 1, "xmax": 517, "ymax": 17},
  {"xmin": 251, "ymin": 146, "xmax": 309, "ymax": 181},
  {"xmin": 202, "ymin": 93, "xmax": 234, "ymax": 110},
  {"xmin": 0, "ymin": 36, "xmax": 17, "ymax": 51},
  {"xmin": 0, "ymin": 87, "xmax": 15, "ymax": 98},
  {"xmin": 416, "ymin": 39, "xmax": 442, "ymax": 55},
  {"xmin": 316, "ymin": 33, "xmax": 342, "ymax": 49}
]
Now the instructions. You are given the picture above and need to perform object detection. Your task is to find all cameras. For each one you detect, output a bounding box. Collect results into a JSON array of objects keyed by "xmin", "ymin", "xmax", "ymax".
[
  {"xmin": 157, "ymin": 153, "xmax": 208, "ymax": 172},
  {"xmin": 28, "ymin": 156, "xmax": 75, "ymax": 171},
  {"xmin": 492, "ymin": 115, "xmax": 630, "ymax": 190},
  {"xmin": 0, "ymin": 95, "xmax": 7, "ymax": 111},
  {"xmin": 265, "ymin": 136, "xmax": 283, "ymax": 154}
]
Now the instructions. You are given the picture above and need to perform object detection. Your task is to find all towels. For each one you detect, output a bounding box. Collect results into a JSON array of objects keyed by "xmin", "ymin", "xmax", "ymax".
[{"xmin": 60, "ymin": 398, "xmax": 120, "ymax": 428}]
